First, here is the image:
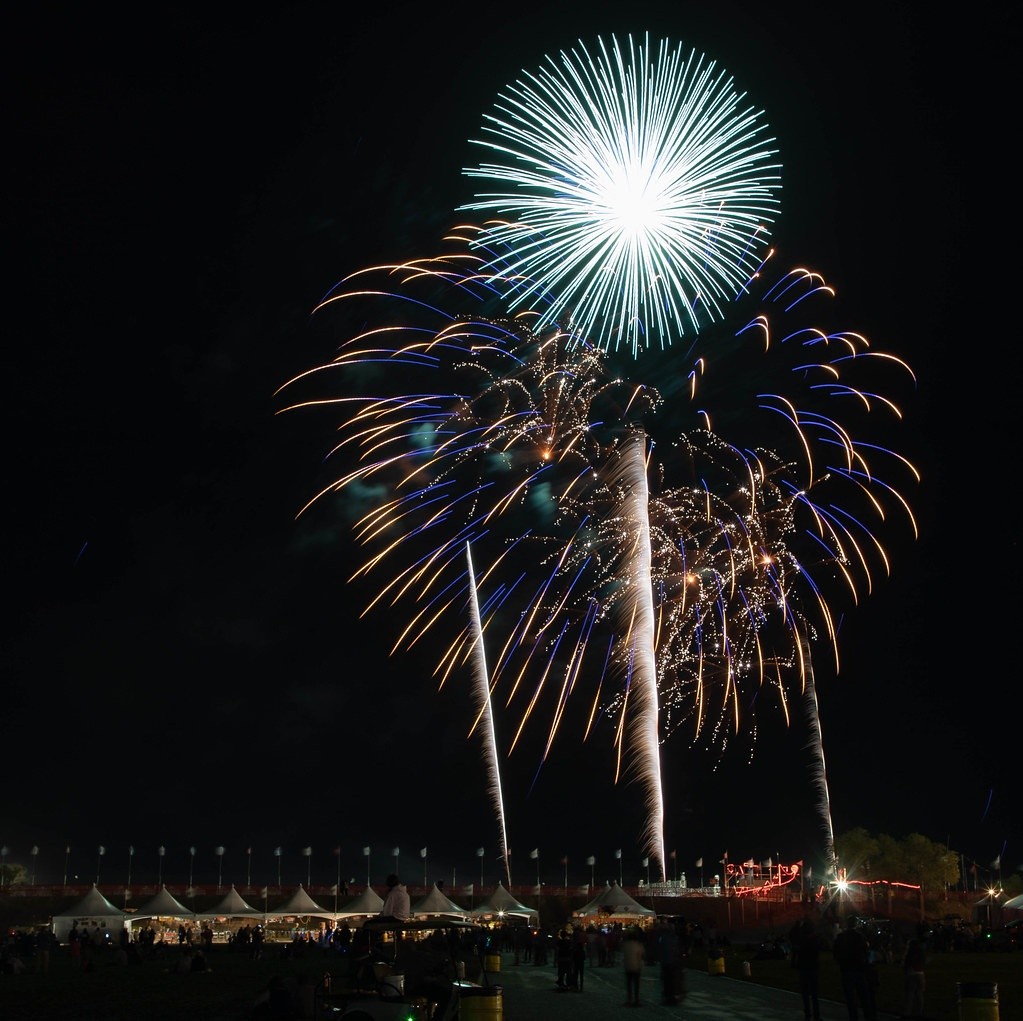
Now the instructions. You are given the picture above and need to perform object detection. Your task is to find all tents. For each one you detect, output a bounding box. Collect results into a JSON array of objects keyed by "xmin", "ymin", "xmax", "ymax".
[{"xmin": 45, "ymin": 880, "xmax": 655, "ymax": 936}]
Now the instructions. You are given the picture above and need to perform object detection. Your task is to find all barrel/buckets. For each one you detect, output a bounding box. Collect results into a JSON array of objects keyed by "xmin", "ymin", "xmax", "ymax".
[
  {"xmin": 383, "ymin": 975, "xmax": 405, "ymax": 996},
  {"xmin": 373, "ymin": 962, "xmax": 387, "ymax": 982}
]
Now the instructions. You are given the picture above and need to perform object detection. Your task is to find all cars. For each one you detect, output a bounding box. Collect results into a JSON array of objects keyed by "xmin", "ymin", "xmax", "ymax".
[
  {"xmin": 982, "ymin": 926, "xmax": 1017, "ymax": 952},
  {"xmin": 249, "ymin": 942, "xmax": 461, "ymax": 1021}
]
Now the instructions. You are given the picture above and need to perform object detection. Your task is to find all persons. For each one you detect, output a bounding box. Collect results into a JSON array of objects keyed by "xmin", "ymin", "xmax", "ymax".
[{"xmin": 0, "ymin": 921, "xmax": 1023, "ymax": 1021}]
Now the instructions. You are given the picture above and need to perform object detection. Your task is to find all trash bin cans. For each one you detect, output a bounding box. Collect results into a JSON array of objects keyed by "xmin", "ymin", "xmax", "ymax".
[
  {"xmin": 486, "ymin": 954, "xmax": 501, "ymax": 973},
  {"xmin": 954, "ymin": 980, "xmax": 1001, "ymax": 1021},
  {"xmin": 704, "ymin": 948, "xmax": 726, "ymax": 976},
  {"xmin": 457, "ymin": 983, "xmax": 504, "ymax": 1021}
]
